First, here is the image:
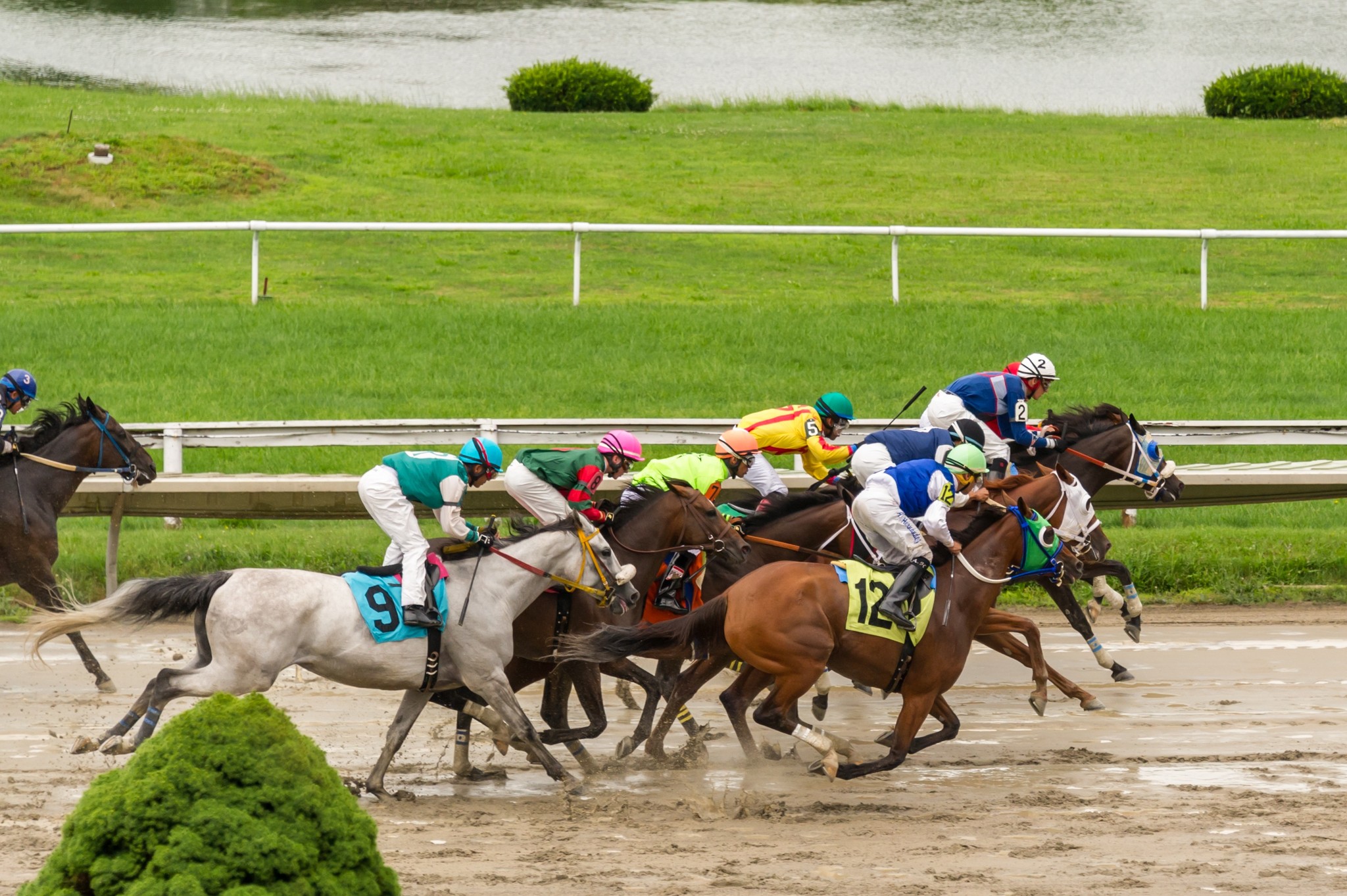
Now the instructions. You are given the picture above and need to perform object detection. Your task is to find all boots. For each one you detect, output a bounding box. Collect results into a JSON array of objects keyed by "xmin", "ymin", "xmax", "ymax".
[
  {"xmin": 653, "ymin": 551, "xmax": 694, "ymax": 615},
  {"xmin": 988, "ymin": 471, "xmax": 1004, "ymax": 481},
  {"xmin": 880, "ymin": 564, "xmax": 923, "ymax": 633}
]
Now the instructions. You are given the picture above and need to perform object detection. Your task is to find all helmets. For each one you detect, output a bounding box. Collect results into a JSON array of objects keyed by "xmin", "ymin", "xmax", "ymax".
[
  {"xmin": 715, "ymin": 428, "xmax": 762, "ymax": 458},
  {"xmin": 947, "ymin": 419, "xmax": 986, "ymax": 452},
  {"xmin": 1018, "ymin": 353, "xmax": 1061, "ymax": 380},
  {"xmin": 598, "ymin": 429, "xmax": 645, "ymax": 462},
  {"xmin": 944, "ymin": 444, "xmax": 990, "ymax": 474},
  {"xmin": 814, "ymin": 392, "xmax": 856, "ymax": 419},
  {"xmin": 459, "ymin": 437, "xmax": 504, "ymax": 472},
  {"xmin": 1, "ymin": 369, "xmax": 40, "ymax": 401},
  {"xmin": 1002, "ymin": 362, "xmax": 1021, "ymax": 375}
]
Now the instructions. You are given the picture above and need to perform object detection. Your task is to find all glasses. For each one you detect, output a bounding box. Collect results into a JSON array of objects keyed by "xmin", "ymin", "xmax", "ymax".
[
  {"xmin": 834, "ymin": 417, "xmax": 849, "ymax": 436},
  {"xmin": 620, "ymin": 455, "xmax": 635, "ymax": 470},
  {"xmin": 20, "ymin": 396, "xmax": 32, "ymax": 407},
  {"xmin": 972, "ymin": 473, "xmax": 983, "ymax": 492},
  {"xmin": 742, "ymin": 455, "xmax": 755, "ymax": 468},
  {"xmin": 1040, "ymin": 378, "xmax": 1051, "ymax": 389},
  {"xmin": 485, "ymin": 465, "xmax": 498, "ymax": 480}
]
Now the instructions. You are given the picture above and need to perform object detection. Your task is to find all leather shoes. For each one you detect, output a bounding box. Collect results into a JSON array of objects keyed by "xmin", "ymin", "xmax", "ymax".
[{"xmin": 403, "ymin": 605, "xmax": 443, "ymax": 627}]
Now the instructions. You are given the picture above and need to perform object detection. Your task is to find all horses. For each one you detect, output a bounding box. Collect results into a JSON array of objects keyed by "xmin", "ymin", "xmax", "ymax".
[
  {"xmin": 532, "ymin": 488, "xmax": 1087, "ymax": 783},
  {"xmin": 424, "ymin": 469, "xmax": 754, "ymax": 783},
  {"xmin": 906, "ymin": 456, "xmax": 1119, "ymax": 723},
  {"xmin": 608, "ymin": 478, "xmax": 881, "ymax": 742},
  {"xmin": 10, "ymin": 505, "xmax": 644, "ymax": 803},
  {"xmin": 822, "ymin": 399, "xmax": 1191, "ymax": 685},
  {"xmin": 0, "ymin": 390, "xmax": 157, "ymax": 696}
]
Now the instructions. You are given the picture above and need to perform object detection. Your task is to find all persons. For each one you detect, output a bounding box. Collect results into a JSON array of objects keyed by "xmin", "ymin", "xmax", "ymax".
[
  {"xmin": 621, "ymin": 429, "xmax": 763, "ymax": 614},
  {"xmin": 851, "ymin": 442, "xmax": 989, "ymax": 630},
  {"xmin": 358, "ymin": 435, "xmax": 504, "ymax": 628},
  {"xmin": 737, "ymin": 391, "xmax": 864, "ymax": 519},
  {"xmin": 0, "ymin": 369, "xmax": 38, "ymax": 455},
  {"xmin": 505, "ymin": 430, "xmax": 645, "ymax": 614},
  {"xmin": 918, "ymin": 353, "xmax": 1067, "ymax": 482},
  {"xmin": 852, "ymin": 418, "xmax": 989, "ymax": 530}
]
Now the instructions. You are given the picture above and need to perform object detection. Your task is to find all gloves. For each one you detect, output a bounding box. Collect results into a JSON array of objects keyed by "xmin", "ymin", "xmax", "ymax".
[
  {"xmin": 1055, "ymin": 436, "xmax": 1076, "ymax": 453},
  {"xmin": 603, "ymin": 505, "xmax": 621, "ymax": 526},
  {"xmin": 854, "ymin": 441, "xmax": 867, "ymax": 449},
  {"xmin": 477, "ymin": 534, "xmax": 494, "ymax": 549},
  {"xmin": 11, "ymin": 442, "xmax": 22, "ymax": 456}
]
